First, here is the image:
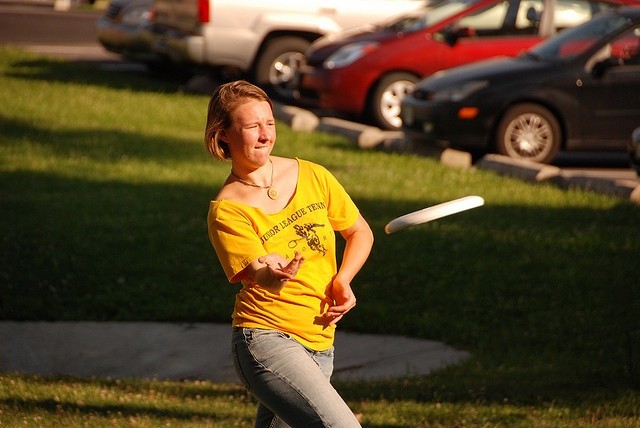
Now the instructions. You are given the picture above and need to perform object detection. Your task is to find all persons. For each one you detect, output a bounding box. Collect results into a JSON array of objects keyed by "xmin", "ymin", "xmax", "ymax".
[{"xmin": 203, "ymin": 80, "xmax": 374, "ymax": 428}]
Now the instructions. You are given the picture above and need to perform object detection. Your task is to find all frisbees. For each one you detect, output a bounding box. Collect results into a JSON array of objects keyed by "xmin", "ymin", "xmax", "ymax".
[{"xmin": 384, "ymin": 194, "xmax": 484, "ymax": 235}]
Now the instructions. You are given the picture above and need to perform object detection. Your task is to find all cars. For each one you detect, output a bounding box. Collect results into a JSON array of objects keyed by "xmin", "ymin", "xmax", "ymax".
[
  {"xmin": 398, "ymin": 5, "xmax": 640, "ymax": 165},
  {"xmin": 291, "ymin": 1, "xmax": 640, "ymax": 131}
]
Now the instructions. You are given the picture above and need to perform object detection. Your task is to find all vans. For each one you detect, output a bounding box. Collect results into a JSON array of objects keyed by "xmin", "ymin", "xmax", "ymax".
[{"xmin": 93, "ymin": 0, "xmax": 432, "ymax": 106}]
